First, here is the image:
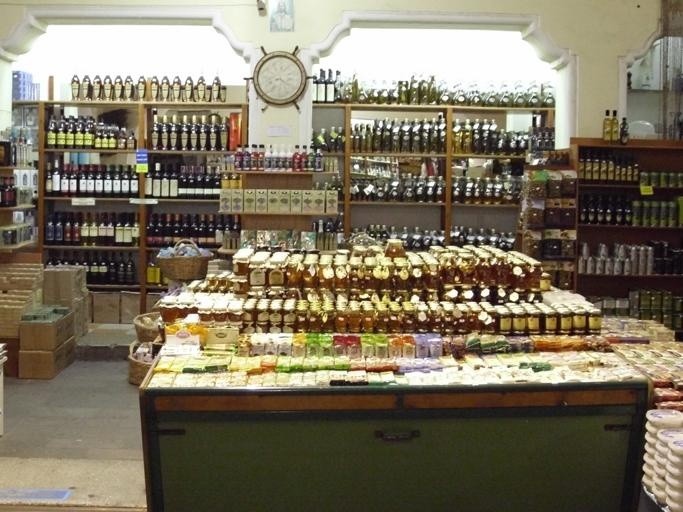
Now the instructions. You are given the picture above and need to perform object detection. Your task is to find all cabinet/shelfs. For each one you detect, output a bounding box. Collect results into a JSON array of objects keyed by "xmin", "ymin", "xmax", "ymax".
[{"xmin": 0, "ymin": 68, "xmax": 682, "ymax": 339}]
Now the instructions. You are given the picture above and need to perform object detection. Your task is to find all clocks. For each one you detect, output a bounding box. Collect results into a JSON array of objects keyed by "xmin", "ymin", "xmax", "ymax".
[{"xmin": 244, "ymin": 45, "xmax": 318, "ymax": 114}]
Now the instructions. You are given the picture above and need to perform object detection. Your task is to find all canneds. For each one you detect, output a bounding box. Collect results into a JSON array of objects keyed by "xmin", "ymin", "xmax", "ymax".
[
  {"xmin": 640, "ymin": 172, "xmax": 683, "ymax": 187},
  {"xmin": 233, "ymin": 238, "xmax": 683, "ymax": 336}
]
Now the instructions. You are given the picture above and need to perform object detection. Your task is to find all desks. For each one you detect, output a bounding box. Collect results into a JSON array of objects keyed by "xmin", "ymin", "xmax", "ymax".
[{"xmin": 136, "ymin": 340, "xmax": 652, "ymax": 512}]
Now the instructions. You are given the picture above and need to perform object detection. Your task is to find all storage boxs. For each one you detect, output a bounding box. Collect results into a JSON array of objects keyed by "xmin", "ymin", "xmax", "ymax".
[
  {"xmin": 127, "ymin": 341, "xmax": 157, "ymax": 385},
  {"xmin": 19, "ymin": 311, "xmax": 74, "ymax": 350},
  {"xmin": 17, "ymin": 337, "xmax": 74, "ymax": 378}
]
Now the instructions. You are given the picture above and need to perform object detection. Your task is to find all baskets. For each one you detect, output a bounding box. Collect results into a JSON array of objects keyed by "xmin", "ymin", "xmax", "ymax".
[
  {"xmin": 132, "ymin": 311, "xmax": 163, "ymax": 343},
  {"xmin": 154, "ymin": 238, "xmax": 214, "ymax": 281},
  {"xmin": 126, "ymin": 339, "xmax": 154, "ymax": 386}
]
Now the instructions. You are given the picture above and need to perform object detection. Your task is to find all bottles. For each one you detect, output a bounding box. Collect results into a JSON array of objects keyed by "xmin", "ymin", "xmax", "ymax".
[
  {"xmin": 46, "ymin": 68, "xmax": 681, "ymax": 287},
  {"xmin": 0, "ymin": 125, "xmax": 38, "ymax": 246}
]
